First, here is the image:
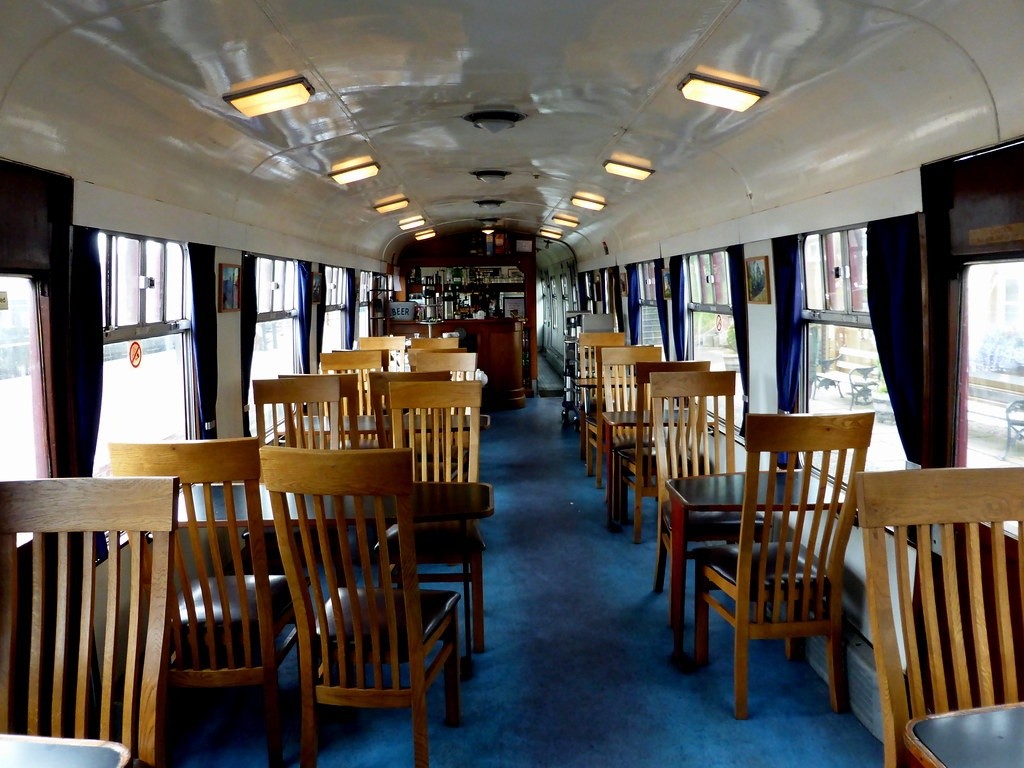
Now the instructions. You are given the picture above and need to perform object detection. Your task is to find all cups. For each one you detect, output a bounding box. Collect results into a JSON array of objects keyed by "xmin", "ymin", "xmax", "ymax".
[
  {"xmin": 456, "ymin": 371, "xmax": 464, "ymax": 381},
  {"xmin": 413, "ymin": 333, "xmax": 419, "ymax": 338},
  {"xmin": 477, "ymin": 310, "xmax": 484, "ymax": 319},
  {"xmin": 443, "ymin": 332, "xmax": 449, "ymax": 339},
  {"xmin": 475, "ymin": 369, "xmax": 484, "ymax": 381},
  {"xmin": 465, "ymin": 371, "xmax": 474, "ymax": 381},
  {"xmin": 451, "ymin": 332, "xmax": 459, "ymax": 338}
]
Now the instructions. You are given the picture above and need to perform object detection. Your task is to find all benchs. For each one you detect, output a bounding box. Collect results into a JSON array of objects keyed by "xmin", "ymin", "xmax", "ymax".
[
  {"xmin": 812, "ymin": 346, "xmax": 882, "ymax": 412},
  {"xmin": 966, "ymin": 382, "xmax": 1024, "ymax": 460}
]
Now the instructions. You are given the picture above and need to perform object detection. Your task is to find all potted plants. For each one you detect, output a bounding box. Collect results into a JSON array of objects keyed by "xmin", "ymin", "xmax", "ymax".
[{"xmin": 450, "ymin": 267, "xmax": 463, "ymax": 284}]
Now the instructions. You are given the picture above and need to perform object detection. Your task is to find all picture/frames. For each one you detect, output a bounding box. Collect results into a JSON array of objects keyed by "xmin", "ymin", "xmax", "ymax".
[
  {"xmin": 218, "ymin": 263, "xmax": 242, "ymax": 313},
  {"xmin": 312, "ymin": 271, "xmax": 323, "ymax": 305},
  {"xmin": 746, "ymin": 255, "xmax": 771, "ymax": 306},
  {"xmin": 619, "ymin": 272, "xmax": 627, "ymax": 295},
  {"xmin": 508, "ymin": 268, "xmax": 524, "ymax": 278},
  {"xmin": 661, "ymin": 268, "xmax": 672, "ymax": 299}
]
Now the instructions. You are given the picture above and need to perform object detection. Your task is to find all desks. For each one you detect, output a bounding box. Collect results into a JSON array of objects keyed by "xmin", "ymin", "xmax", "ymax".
[
  {"xmin": 665, "ymin": 468, "xmax": 847, "ymax": 655},
  {"xmin": 571, "ymin": 378, "xmax": 639, "ymax": 462},
  {"xmin": 1, "ymin": 733, "xmax": 132, "ymax": 768},
  {"xmin": 601, "ymin": 408, "xmax": 714, "ymax": 531},
  {"xmin": 178, "ymin": 481, "xmax": 494, "ymax": 678},
  {"xmin": 276, "ymin": 414, "xmax": 492, "ymax": 549},
  {"xmin": 904, "ymin": 702, "xmax": 1024, "ymax": 768}
]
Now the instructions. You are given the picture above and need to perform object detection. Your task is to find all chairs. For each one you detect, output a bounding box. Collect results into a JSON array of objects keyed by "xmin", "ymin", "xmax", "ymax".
[
  {"xmin": 578, "ymin": 332, "xmax": 1024, "ymax": 768},
  {"xmin": 0, "ymin": 336, "xmax": 484, "ymax": 768}
]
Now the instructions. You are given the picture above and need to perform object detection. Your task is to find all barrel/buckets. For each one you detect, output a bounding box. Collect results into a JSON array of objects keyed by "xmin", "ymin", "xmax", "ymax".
[
  {"xmin": 425, "ymin": 296, "xmax": 436, "ymax": 319},
  {"xmin": 444, "ymin": 295, "xmax": 454, "ymax": 320}
]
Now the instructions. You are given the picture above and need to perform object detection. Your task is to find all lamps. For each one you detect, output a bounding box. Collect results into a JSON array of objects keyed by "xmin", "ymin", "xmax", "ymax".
[{"xmin": 222, "ymin": 71, "xmax": 769, "ymax": 241}]
